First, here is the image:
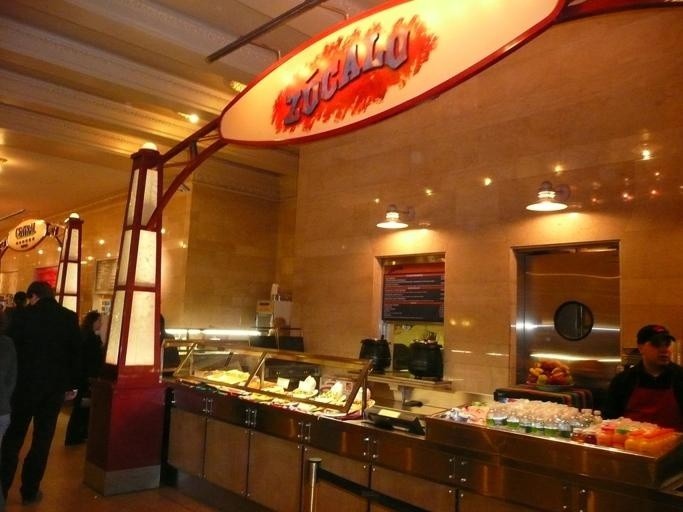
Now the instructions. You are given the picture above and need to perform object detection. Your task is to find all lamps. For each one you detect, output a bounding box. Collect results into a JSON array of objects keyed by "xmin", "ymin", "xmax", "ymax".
[
  {"xmin": 376, "ymin": 204, "xmax": 415, "ymax": 229},
  {"xmin": 525, "ymin": 181, "xmax": 570, "ymax": 211}
]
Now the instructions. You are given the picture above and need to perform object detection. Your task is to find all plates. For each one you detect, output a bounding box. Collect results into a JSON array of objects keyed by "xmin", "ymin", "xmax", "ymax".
[
  {"xmin": 248, "ymin": 385, "xmax": 376, "ymax": 417},
  {"xmin": 526, "ymin": 381, "xmax": 575, "ymax": 392}
]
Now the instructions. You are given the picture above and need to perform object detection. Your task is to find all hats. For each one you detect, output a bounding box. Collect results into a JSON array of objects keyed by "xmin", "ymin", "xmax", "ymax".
[{"xmin": 637, "ymin": 324, "xmax": 676, "ymax": 344}]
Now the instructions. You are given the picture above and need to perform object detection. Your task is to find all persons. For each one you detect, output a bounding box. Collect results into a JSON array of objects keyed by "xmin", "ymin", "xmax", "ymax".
[
  {"xmin": 608, "ymin": 326, "xmax": 683, "ymax": 465},
  {"xmin": 0, "ymin": 280, "xmax": 80, "ymax": 506},
  {"xmin": 0, "ymin": 281, "xmax": 103, "ymax": 450},
  {"xmin": 159, "ymin": 313, "xmax": 181, "ymax": 485}
]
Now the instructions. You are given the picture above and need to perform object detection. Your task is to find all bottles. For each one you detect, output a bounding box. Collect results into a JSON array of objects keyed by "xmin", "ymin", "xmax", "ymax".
[
  {"xmin": 270, "ymin": 283, "xmax": 280, "ymax": 301},
  {"xmin": 485, "ymin": 397, "xmax": 678, "ymax": 460}
]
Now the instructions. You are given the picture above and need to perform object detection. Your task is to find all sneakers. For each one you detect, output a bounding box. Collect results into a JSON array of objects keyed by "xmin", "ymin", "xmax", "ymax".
[{"xmin": 22, "ymin": 490, "xmax": 42, "ymax": 505}]
